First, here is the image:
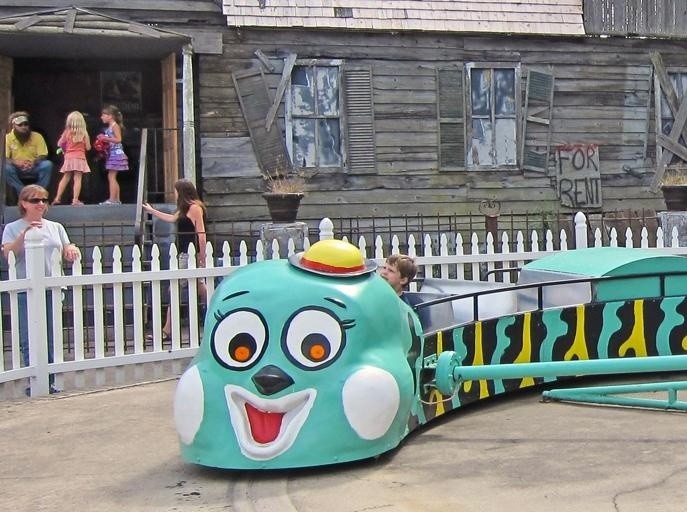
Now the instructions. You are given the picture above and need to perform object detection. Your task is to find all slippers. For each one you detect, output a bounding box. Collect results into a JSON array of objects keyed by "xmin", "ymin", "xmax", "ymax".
[{"xmin": 145, "ymin": 331, "xmax": 171, "ymax": 342}]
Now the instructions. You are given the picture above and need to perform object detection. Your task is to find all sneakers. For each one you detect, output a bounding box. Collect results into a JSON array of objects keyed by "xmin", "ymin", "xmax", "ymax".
[
  {"xmin": 72, "ymin": 202, "xmax": 84, "ymax": 206},
  {"xmin": 99, "ymin": 200, "xmax": 121, "ymax": 206},
  {"xmin": 25, "ymin": 383, "xmax": 64, "ymax": 397},
  {"xmin": 50, "ymin": 200, "xmax": 60, "ymax": 206}
]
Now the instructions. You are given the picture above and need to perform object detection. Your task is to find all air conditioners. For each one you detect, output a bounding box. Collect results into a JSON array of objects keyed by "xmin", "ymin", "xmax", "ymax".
[{"xmin": 259, "ymin": 155, "xmax": 305, "ymax": 222}]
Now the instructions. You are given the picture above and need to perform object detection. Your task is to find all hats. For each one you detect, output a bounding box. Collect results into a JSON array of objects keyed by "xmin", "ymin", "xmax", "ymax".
[{"xmin": 12, "ymin": 116, "xmax": 28, "ymax": 124}]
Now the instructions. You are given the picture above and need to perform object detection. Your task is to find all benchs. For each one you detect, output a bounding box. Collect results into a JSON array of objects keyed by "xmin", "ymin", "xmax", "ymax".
[{"xmin": 402, "ymin": 269, "xmax": 592, "ymax": 334}]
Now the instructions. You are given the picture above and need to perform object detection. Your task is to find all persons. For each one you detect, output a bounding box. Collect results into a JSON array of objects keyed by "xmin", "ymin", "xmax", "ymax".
[
  {"xmin": 381, "ymin": 254, "xmax": 431, "ymax": 334},
  {"xmin": 4, "ymin": 110, "xmax": 54, "ymax": 195},
  {"xmin": 95, "ymin": 104, "xmax": 130, "ymax": 204},
  {"xmin": 1, "ymin": 184, "xmax": 80, "ymax": 397},
  {"xmin": 49, "ymin": 110, "xmax": 91, "ymax": 205},
  {"xmin": 142, "ymin": 177, "xmax": 206, "ymax": 341}
]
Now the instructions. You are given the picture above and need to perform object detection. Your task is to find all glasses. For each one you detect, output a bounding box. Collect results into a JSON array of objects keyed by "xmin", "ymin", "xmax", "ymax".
[{"xmin": 26, "ymin": 199, "xmax": 47, "ymax": 203}]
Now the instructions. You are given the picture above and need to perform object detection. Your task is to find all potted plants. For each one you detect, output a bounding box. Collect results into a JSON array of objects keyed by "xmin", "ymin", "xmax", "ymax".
[{"xmin": 657, "ymin": 167, "xmax": 687, "ymax": 212}]
